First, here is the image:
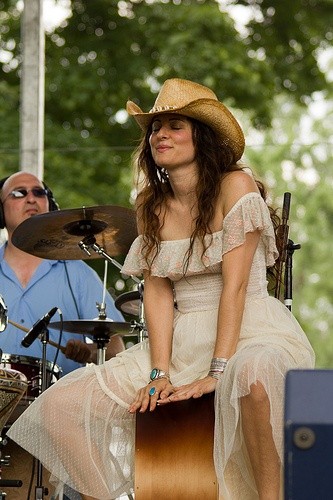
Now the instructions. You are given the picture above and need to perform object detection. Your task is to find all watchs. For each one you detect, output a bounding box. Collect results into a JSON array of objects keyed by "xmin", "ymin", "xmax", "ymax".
[{"xmin": 150, "ymin": 368, "xmax": 170, "ymax": 380}]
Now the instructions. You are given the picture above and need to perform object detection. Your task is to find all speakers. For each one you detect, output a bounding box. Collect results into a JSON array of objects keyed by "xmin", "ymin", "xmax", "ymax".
[{"xmin": 283, "ymin": 369, "xmax": 333, "ymax": 500}]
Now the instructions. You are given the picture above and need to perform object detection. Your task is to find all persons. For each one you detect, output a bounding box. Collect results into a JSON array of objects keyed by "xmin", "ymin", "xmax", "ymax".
[
  {"xmin": 6, "ymin": 79, "xmax": 315, "ymax": 500},
  {"xmin": 0, "ymin": 172, "xmax": 126, "ymax": 382}
]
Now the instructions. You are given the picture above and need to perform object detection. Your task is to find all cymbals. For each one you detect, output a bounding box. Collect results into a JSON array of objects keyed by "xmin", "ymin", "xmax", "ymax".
[
  {"xmin": 115, "ymin": 290, "xmax": 142, "ymax": 316},
  {"xmin": 11, "ymin": 206, "xmax": 139, "ymax": 260},
  {"xmin": 47, "ymin": 318, "xmax": 139, "ymax": 337}
]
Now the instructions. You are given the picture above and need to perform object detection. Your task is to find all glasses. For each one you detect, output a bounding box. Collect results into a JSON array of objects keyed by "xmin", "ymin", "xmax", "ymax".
[{"xmin": 3, "ymin": 188, "xmax": 49, "ymax": 204}]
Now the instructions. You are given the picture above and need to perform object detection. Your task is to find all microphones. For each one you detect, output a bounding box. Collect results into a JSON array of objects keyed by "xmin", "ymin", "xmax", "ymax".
[{"xmin": 21, "ymin": 307, "xmax": 58, "ymax": 348}]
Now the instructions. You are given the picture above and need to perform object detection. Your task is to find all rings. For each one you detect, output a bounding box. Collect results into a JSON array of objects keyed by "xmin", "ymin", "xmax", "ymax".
[{"xmin": 150, "ymin": 387, "xmax": 159, "ymax": 396}]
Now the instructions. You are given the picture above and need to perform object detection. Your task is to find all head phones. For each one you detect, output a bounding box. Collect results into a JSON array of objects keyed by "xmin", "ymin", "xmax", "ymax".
[{"xmin": 0, "ymin": 176, "xmax": 60, "ymax": 229}]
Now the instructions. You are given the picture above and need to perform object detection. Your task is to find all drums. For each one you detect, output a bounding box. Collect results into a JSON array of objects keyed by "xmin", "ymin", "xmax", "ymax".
[
  {"xmin": 0, "ymin": 368, "xmax": 27, "ymax": 437},
  {"xmin": 0, "ymin": 352, "xmax": 62, "ymax": 399}
]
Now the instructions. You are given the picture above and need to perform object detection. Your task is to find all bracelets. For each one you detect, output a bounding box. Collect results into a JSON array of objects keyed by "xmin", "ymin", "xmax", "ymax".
[
  {"xmin": 208, "ymin": 373, "xmax": 220, "ymax": 380},
  {"xmin": 210, "ymin": 358, "xmax": 227, "ymax": 372}
]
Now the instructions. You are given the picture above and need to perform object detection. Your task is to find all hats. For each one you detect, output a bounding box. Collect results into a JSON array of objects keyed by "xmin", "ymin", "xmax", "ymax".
[{"xmin": 126, "ymin": 78, "xmax": 245, "ymax": 163}]
